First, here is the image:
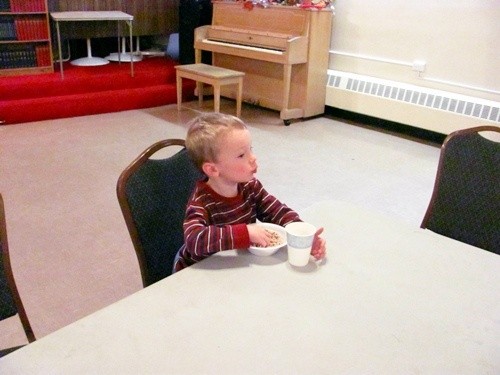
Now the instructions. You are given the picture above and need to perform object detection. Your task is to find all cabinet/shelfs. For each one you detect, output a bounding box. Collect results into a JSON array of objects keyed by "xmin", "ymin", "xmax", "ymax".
[{"xmin": 0, "ymin": 0, "xmax": 54, "ymax": 77}]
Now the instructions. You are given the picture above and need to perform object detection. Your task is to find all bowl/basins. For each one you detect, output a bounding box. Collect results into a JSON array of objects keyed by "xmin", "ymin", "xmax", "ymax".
[{"xmin": 247, "ymin": 222, "xmax": 288, "ymax": 256}]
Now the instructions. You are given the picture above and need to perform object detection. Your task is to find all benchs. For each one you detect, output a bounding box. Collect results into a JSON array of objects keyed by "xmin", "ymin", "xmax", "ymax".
[{"xmin": 176, "ymin": 61, "xmax": 242, "ymax": 118}]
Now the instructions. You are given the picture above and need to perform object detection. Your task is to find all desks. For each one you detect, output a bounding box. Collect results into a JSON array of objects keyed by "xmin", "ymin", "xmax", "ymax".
[
  {"xmin": 50, "ymin": 10, "xmax": 135, "ymax": 80},
  {"xmin": 0, "ymin": 198, "xmax": 500, "ymax": 375}
]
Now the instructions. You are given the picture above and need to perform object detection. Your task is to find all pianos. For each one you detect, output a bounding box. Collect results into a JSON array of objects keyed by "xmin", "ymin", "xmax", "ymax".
[{"xmin": 194, "ymin": 0, "xmax": 332, "ymax": 125}]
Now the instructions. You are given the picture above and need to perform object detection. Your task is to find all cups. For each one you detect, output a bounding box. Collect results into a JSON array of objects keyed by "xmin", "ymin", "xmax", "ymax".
[{"xmin": 285, "ymin": 221, "xmax": 317, "ymax": 267}]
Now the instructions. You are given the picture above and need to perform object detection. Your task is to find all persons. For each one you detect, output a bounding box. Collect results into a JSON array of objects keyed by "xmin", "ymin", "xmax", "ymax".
[{"xmin": 171, "ymin": 112, "xmax": 325, "ymax": 274}]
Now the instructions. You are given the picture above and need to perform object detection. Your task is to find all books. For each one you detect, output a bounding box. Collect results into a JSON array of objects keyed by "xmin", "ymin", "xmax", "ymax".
[
  {"xmin": 0, "ymin": 0, "xmax": 45, "ymax": 13},
  {"xmin": 0, "ymin": 44, "xmax": 51, "ymax": 69},
  {"xmin": 0, "ymin": 19, "xmax": 48, "ymax": 41}
]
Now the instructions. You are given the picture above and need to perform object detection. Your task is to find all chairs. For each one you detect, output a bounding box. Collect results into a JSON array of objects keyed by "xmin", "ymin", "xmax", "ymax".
[
  {"xmin": 0, "ymin": 193, "xmax": 37, "ymax": 358},
  {"xmin": 420, "ymin": 126, "xmax": 500, "ymax": 256},
  {"xmin": 117, "ymin": 138, "xmax": 206, "ymax": 290}
]
